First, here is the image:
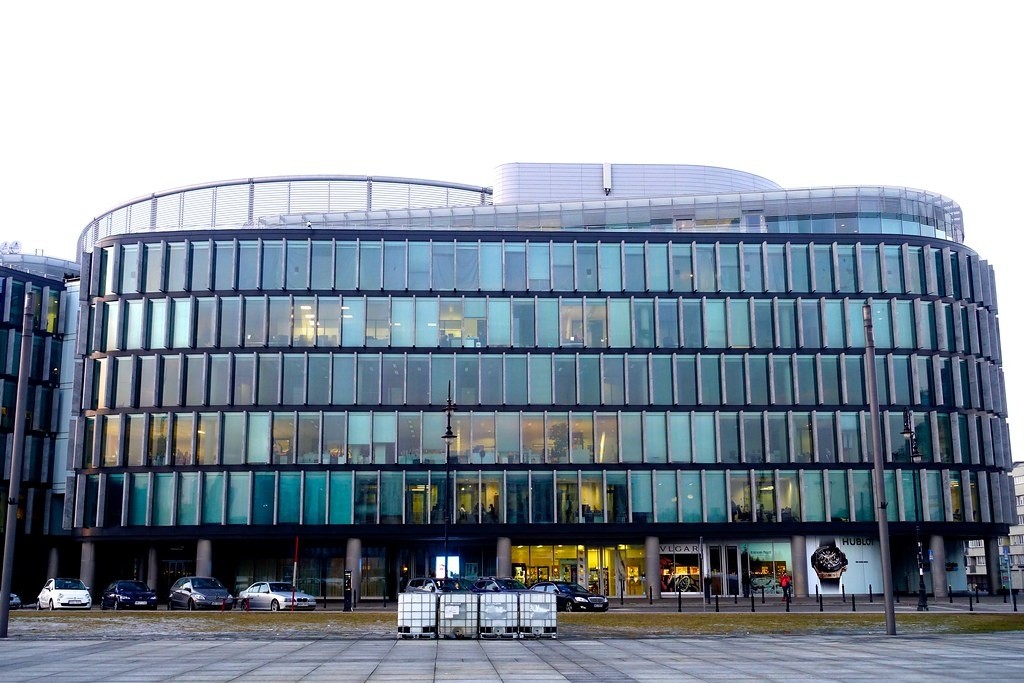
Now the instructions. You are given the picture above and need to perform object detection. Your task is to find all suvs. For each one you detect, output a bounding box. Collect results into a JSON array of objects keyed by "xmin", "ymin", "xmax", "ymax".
[
  {"xmin": 166, "ymin": 576, "xmax": 234, "ymax": 612},
  {"xmin": 403, "ymin": 575, "xmax": 533, "ymax": 596}
]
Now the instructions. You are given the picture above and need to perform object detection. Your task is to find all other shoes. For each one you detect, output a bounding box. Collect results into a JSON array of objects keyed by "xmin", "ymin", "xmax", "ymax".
[{"xmin": 781, "ymin": 598, "xmax": 785, "ymax": 601}]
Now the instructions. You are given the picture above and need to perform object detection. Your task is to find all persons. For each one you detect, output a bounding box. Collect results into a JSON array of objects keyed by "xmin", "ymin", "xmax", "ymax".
[
  {"xmin": 472, "ymin": 503, "xmax": 484, "ymax": 524},
  {"xmin": 460, "ymin": 504, "xmax": 467, "ymax": 521},
  {"xmin": 488, "ymin": 504, "xmax": 495, "ymax": 513},
  {"xmin": 780, "ymin": 571, "xmax": 791, "ymax": 601}
]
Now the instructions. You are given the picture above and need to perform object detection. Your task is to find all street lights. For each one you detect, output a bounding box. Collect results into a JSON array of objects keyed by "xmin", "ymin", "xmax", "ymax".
[
  {"xmin": 900, "ymin": 405, "xmax": 930, "ymax": 611},
  {"xmin": 440, "ymin": 379, "xmax": 458, "ymax": 579}
]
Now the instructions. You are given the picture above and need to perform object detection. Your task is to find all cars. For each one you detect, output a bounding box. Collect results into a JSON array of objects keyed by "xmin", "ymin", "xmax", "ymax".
[
  {"xmin": 35, "ymin": 577, "xmax": 92, "ymax": 611},
  {"xmin": 529, "ymin": 580, "xmax": 609, "ymax": 612},
  {"xmin": 237, "ymin": 581, "xmax": 317, "ymax": 611},
  {"xmin": 100, "ymin": 580, "xmax": 159, "ymax": 610}
]
